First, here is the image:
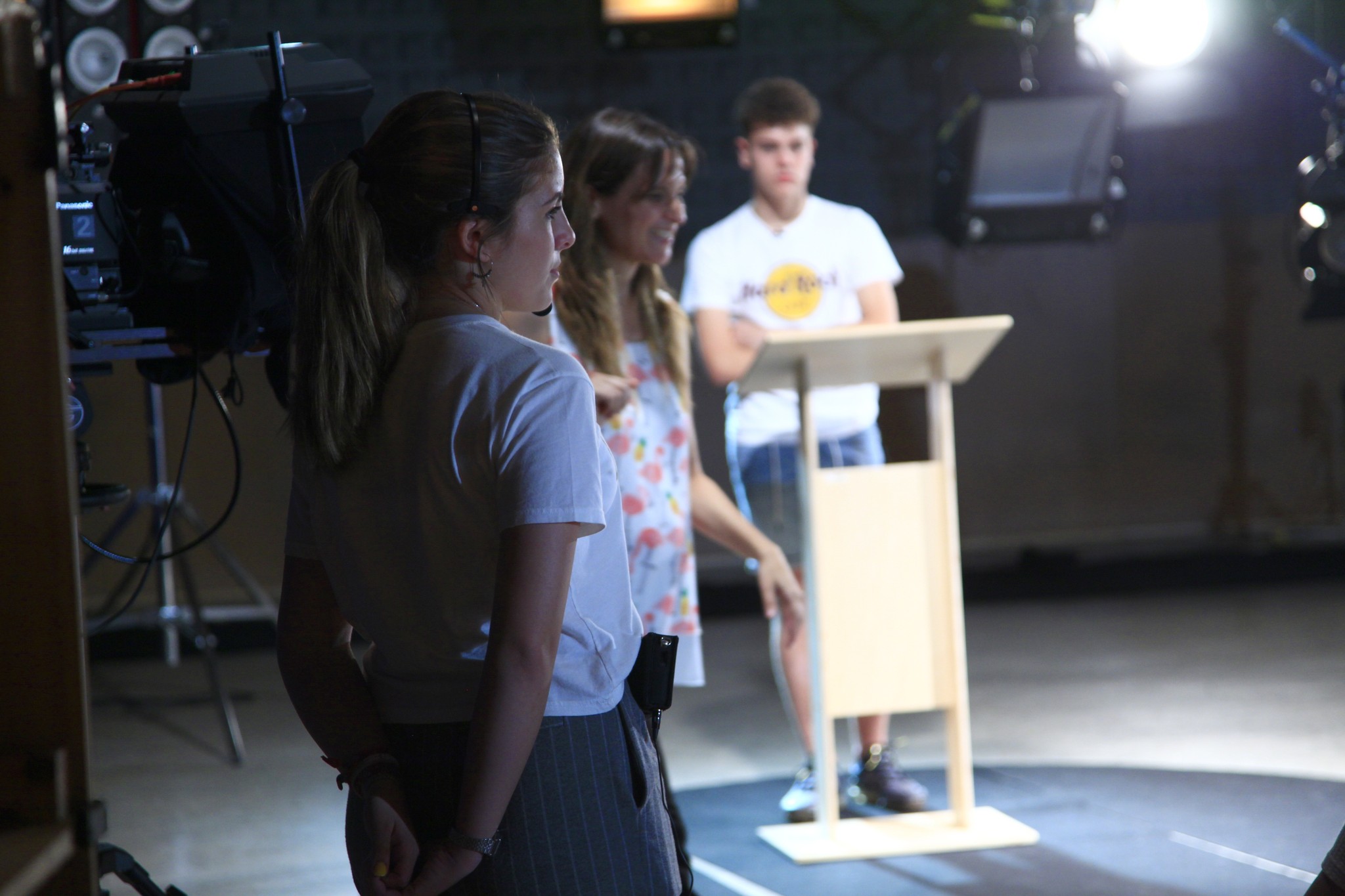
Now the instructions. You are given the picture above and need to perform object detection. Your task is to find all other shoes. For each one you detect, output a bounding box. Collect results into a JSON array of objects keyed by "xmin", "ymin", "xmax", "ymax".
[
  {"xmin": 849, "ymin": 740, "xmax": 931, "ymax": 812},
  {"xmin": 780, "ymin": 763, "xmax": 843, "ymax": 819}
]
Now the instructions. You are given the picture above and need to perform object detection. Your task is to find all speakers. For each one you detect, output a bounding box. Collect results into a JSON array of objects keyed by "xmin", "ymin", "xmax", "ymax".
[{"xmin": 116, "ymin": 39, "xmax": 367, "ymax": 361}]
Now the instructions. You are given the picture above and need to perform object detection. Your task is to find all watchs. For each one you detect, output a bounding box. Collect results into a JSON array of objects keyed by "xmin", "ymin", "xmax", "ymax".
[{"xmin": 448, "ymin": 830, "xmax": 502, "ymax": 858}]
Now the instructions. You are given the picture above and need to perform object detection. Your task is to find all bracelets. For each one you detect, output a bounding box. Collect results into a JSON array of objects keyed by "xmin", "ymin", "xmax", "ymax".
[{"xmin": 321, "ymin": 747, "xmax": 402, "ymax": 790}]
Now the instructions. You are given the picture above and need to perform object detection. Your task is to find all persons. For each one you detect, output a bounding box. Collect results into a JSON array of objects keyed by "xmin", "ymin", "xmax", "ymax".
[
  {"xmin": 679, "ymin": 77, "xmax": 928, "ymax": 821},
  {"xmin": 500, "ymin": 107, "xmax": 806, "ymax": 896},
  {"xmin": 276, "ymin": 91, "xmax": 683, "ymax": 896}
]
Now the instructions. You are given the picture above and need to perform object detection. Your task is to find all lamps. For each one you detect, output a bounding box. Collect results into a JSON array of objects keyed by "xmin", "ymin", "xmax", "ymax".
[
  {"xmin": 1290, "ymin": 106, "xmax": 1345, "ymax": 320},
  {"xmin": 936, "ymin": 30, "xmax": 1136, "ymax": 249},
  {"xmin": 581, "ymin": 0, "xmax": 744, "ymax": 58},
  {"xmin": 33, "ymin": 0, "xmax": 282, "ymax": 767}
]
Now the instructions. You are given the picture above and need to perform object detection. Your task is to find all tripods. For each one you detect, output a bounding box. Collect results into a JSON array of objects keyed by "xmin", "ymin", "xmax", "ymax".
[{"xmin": 76, "ymin": 382, "xmax": 287, "ymax": 766}]
